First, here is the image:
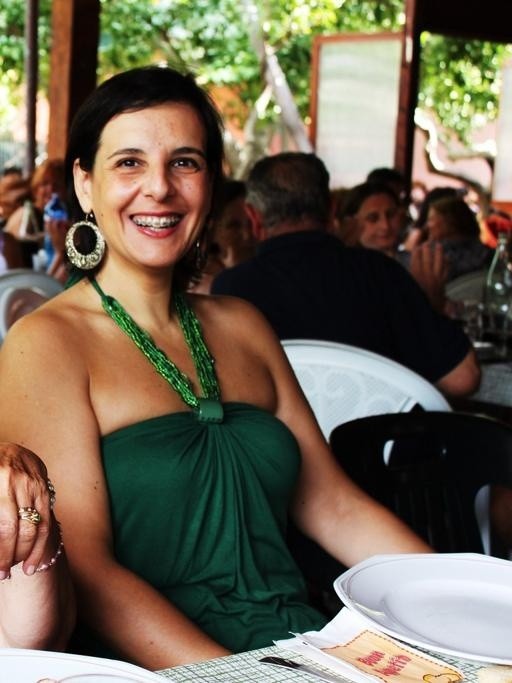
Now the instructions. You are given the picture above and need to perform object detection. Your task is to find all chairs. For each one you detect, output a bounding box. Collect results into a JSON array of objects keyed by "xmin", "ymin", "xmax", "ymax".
[
  {"xmin": 0, "ymin": 268, "xmax": 66, "ymax": 348},
  {"xmin": 276, "ymin": 340, "xmax": 512, "ymax": 562}
]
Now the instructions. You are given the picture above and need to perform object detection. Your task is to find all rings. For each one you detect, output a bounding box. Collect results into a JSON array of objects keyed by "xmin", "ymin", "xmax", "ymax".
[{"xmin": 16, "ymin": 507, "xmax": 40, "ymax": 524}]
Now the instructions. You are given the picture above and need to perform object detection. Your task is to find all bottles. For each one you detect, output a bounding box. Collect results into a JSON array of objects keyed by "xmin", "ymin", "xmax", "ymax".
[{"xmin": 484, "ymin": 231, "xmax": 512, "ymax": 336}]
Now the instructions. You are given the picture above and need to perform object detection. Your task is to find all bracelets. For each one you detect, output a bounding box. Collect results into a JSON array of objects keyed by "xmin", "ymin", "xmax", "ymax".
[{"xmin": 36, "ymin": 481, "xmax": 64, "ymax": 571}]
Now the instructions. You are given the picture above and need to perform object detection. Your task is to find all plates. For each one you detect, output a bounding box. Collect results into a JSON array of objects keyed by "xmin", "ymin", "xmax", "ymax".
[{"xmin": 333, "ymin": 551, "xmax": 512, "ymax": 666}]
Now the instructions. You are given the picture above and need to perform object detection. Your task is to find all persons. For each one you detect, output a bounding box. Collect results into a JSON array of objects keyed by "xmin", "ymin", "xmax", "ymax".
[
  {"xmin": 0, "ymin": 66, "xmax": 431, "ymax": 671},
  {"xmin": 212, "ymin": 151, "xmax": 482, "ymax": 407},
  {"xmin": 0, "ymin": 442, "xmax": 72, "ymax": 652},
  {"xmin": 0, "ymin": 159, "xmax": 505, "ymax": 293}
]
